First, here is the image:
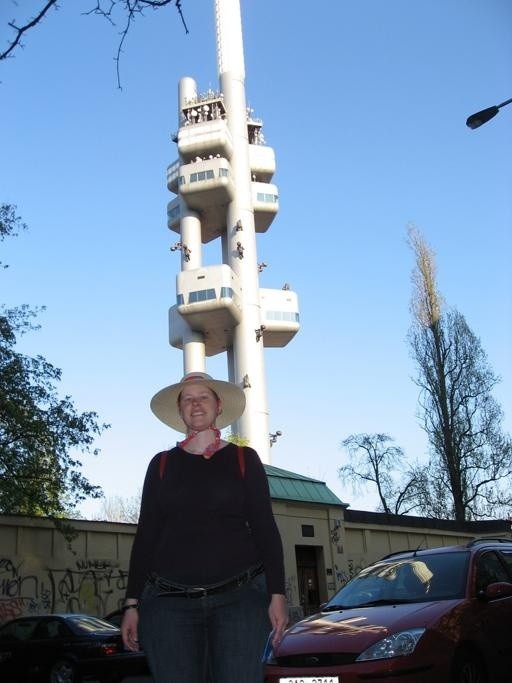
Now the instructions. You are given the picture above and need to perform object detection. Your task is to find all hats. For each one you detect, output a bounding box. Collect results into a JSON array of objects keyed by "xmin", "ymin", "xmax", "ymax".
[{"xmin": 148, "ymin": 369, "xmax": 247, "ymax": 432}]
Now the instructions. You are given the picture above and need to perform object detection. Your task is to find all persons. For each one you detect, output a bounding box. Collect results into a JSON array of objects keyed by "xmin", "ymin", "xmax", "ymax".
[{"xmin": 119, "ymin": 372, "xmax": 291, "ymax": 682}]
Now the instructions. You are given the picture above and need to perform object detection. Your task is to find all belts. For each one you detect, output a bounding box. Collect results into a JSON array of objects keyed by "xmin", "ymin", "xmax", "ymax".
[{"xmin": 146, "ymin": 562, "xmax": 269, "ymax": 602}]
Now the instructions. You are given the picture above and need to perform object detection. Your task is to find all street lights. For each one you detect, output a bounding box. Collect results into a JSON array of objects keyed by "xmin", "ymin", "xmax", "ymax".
[{"xmin": 465, "ymin": 94, "xmax": 512, "ymax": 132}]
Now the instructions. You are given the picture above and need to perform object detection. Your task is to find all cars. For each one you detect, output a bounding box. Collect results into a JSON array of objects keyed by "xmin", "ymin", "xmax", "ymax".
[
  {"xmin": 102, "ymin": 607, "xmax": 129, "ymax": 630},
  {"xmin": 1, "ymin": 616, "xmax": 146, "ymax": 682}
]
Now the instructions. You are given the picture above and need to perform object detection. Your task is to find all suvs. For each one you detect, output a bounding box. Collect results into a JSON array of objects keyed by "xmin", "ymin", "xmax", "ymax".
[{"xmin": 263, "ymin": 537, "xmax": 512, "ymax": 682}]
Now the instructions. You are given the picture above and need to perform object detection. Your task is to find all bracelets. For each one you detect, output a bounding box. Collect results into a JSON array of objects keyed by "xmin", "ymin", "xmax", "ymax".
[{"xmin": 123, "ymin": 601, "xmax": 141, "ymax": 614}]
